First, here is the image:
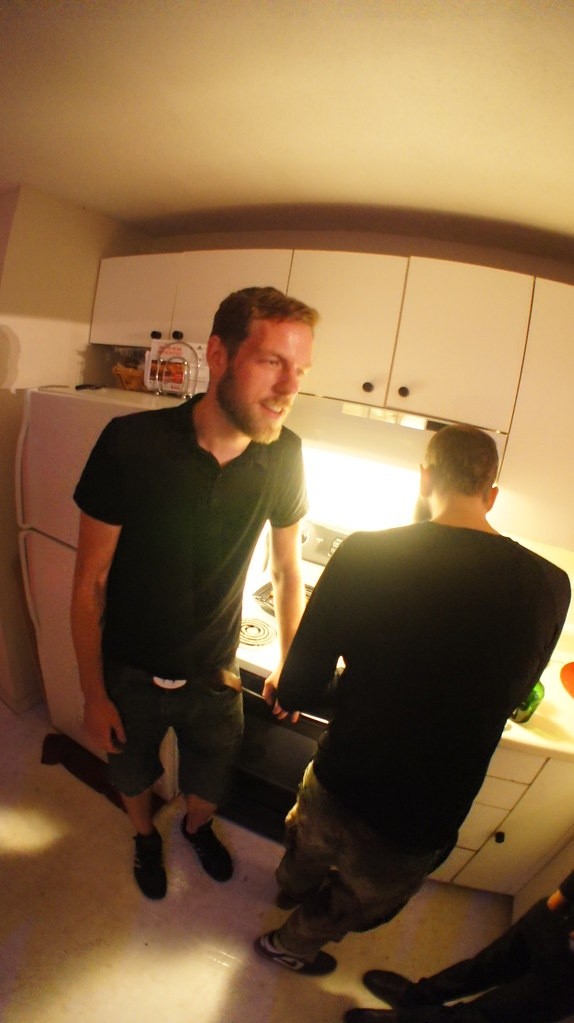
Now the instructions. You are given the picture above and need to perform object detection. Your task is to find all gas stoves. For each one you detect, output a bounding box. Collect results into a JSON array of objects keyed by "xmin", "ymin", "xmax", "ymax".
[{"xmin": 231, "ymin": 521, "xmax": 351, "ymax": 702}]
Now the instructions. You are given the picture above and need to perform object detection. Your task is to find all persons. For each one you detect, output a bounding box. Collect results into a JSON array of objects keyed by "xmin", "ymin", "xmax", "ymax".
[
  {"xmin": 69, "ymin": 288, "xmax": 321, "ymax": 899},
  {"xmin": 254, "ymin": 426, "xmax": 571, "ymax": 976},
  {"xmin": 343, "ymin": 872, "xmax": 574, "ymax": 1023}
]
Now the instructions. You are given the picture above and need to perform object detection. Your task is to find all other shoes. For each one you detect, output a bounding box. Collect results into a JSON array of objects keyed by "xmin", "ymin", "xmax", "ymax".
[
  {"xmin": 180, "ymin": 814, "xmax": 234, "ymax": 882},
  {"xmin": 133, "ymin": 828, "xmax": 167, "ymax": 899},
  {"xmin": 363, "ymin": 969, "xmax": 415, "ymax": 1007},
  {"xmin": 344, "ymin": 1008, "xmax": 399, "ymax": 1023}
]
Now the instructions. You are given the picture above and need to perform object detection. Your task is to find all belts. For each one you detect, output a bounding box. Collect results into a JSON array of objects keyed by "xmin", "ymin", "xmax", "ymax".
[{"xmin": 119, "ymin": 665, "xmax": 242, "ymax": 693}]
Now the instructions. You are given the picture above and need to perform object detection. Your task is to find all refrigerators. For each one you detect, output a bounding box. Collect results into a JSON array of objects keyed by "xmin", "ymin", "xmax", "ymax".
[{"xmin": 14, "ymin": 384, "xmax": 191, "ymax": 803}]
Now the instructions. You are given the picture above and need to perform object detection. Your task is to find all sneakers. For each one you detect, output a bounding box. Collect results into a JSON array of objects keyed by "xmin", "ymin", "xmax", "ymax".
[
  {"xmin": 277, "ymin": 890, "xmax": 299, "ymax": 911},
  {"xmin": 254, "ymin": 930, "xmax": 337, "ymax": 976}
]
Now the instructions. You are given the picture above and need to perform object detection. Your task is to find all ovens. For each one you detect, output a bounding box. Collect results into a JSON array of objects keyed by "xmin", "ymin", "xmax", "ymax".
[{"xmin": 181, "ymin": 666, "xmax": 330, "ymax": 846}]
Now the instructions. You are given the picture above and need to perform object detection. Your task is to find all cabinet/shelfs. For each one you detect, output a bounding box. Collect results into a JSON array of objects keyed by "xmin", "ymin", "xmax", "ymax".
[
  {"xmin": 424, "ymin": 747, "xmax": 574, "ymax": 904},
  {"xmin": 87, "ymin": 249, "xmax": 574, "ymax": 551}
]
